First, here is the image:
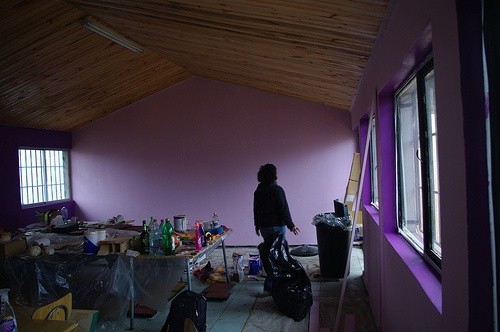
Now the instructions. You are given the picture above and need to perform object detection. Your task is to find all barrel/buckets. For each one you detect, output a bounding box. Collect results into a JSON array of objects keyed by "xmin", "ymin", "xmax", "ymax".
[{"xmin": 174, "ymin": 215, "xmax": 187, "ymax": 232}]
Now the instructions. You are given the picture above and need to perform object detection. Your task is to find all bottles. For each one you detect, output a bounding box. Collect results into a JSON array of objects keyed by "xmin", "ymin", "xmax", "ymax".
[
  {"xmin": 211, "ymin": 212, "xmax": 219, "ymax": 229},
  {"xmin": 61, "ymin": 205, "xmax": 68, "ymax": 225},
  {"xmin": 140, "ymin": 217, "xmax": 176, "ymax": 257},
  {"xmin": 194, "ymin": 222, "xmax": 207, "ymax": 252}
]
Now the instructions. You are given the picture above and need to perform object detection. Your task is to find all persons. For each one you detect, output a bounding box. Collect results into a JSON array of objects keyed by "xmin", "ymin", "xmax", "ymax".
[{"xmin": 254, "ymin": 163, "xmax": 301, "ymax": 296}]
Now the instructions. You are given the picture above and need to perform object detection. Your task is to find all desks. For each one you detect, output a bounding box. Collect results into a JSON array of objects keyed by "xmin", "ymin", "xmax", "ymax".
[{"xmin": 0, "ymin": 221, "xmax": 232, "ymax": 332}]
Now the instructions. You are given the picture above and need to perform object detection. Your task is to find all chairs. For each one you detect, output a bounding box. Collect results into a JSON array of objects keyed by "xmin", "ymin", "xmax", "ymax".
[{"xmin": 32, "ymin": 292, "xmax": 73, "ymax": 322}]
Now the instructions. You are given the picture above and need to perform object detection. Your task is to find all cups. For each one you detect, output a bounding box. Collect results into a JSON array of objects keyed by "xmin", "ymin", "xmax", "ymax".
[{"xmin": 0, "ymin": 288, "xmax": 18, "ymax": 332}]
